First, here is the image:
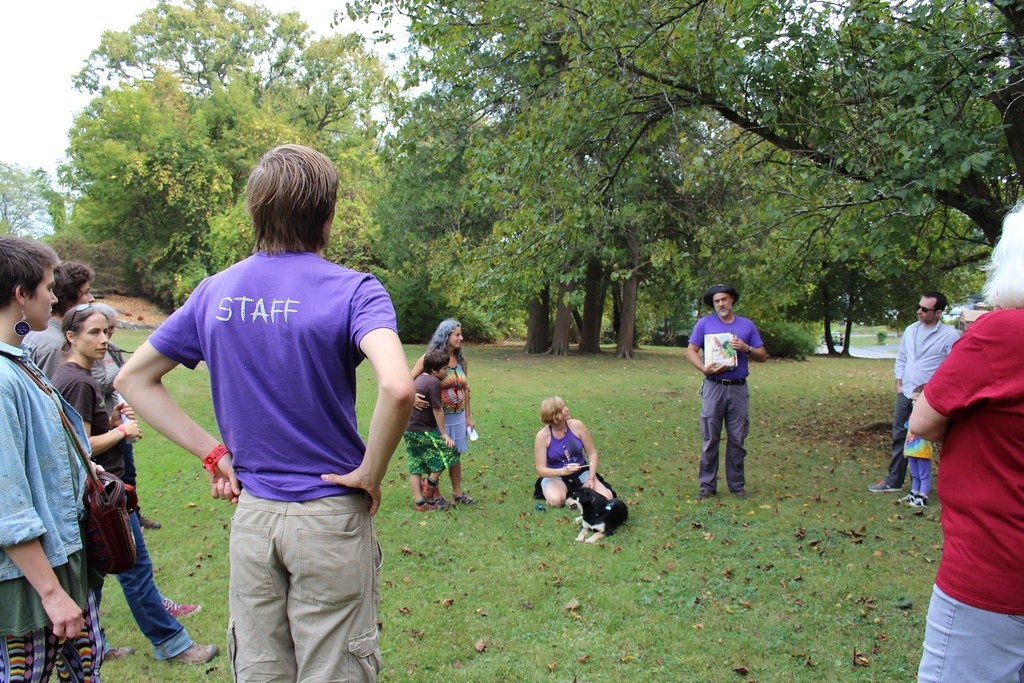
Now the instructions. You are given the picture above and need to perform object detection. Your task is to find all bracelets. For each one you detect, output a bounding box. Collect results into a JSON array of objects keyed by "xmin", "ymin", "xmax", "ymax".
[
  {"xmin": 117, "ymin": 426, "xmax": 127, "ymax": 439},
  {"xmin": 588, "ymin": 478, "xmax": 595, "ymax": 480},
  {"xmin": 202, "ymin": 443, "xmax": 229, "ymax": 477}
]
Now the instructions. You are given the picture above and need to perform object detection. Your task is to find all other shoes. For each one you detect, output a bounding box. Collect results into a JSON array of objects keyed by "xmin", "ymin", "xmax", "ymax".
[
  {"xmin": 737, "ymin": 490, "xmax": 749, "ymax": 498},
  {"xmin": 695, "ymin": 489, "xmax": 716, "ymax": 499},
  {"xmin": 140, "ymin": 517, "xmax": 162, "ymax": 529}
]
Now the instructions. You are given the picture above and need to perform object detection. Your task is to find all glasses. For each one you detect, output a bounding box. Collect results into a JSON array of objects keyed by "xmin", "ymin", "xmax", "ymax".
[
  {"xmin": 69, "ymin": 303, "xmax": 89, "ymax": 330},
  {"xmin": 916, "ymin": 304, "xmax": 936, "ymax": 312}
]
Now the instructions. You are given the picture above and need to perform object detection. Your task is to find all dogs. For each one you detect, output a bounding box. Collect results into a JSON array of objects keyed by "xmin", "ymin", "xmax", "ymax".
[{"xmin": 564, "ymin": 487, "xmax": 628, "ymax": 544}]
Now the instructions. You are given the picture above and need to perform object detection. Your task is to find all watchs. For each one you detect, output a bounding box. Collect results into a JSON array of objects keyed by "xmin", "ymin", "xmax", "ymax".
[{"xmin": 745, "ymin": 345, "xmax": 752, "ymax": 354}]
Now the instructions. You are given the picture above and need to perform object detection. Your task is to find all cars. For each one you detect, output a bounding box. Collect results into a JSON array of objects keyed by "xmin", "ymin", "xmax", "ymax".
[{"xmin": 821, "ymin": 332, "xmax": 843, "ymax": 345}]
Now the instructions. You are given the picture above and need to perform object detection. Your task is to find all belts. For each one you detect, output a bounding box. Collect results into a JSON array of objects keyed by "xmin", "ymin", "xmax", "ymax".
[{"xmin": 706, "ymin": 376, "xmax": 745, "ymax": 385}]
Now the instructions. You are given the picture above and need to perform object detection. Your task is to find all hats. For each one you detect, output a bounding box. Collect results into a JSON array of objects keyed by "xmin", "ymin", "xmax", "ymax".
[{"xmin": 703, "ymin": 283, "xmax": 740, "ymax": 309}]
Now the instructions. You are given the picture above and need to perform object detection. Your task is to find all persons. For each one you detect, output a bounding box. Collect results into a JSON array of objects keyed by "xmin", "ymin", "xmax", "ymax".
[
  {"xmin": 0, "ymin": 237, "xmax": 105, "ymax": 683},
  {"xmin": 114, "ymin": 143, "xmax": 416, "ymax": 683},
  {"xmin": 535, "ymin": 396, "xmax": 613, "ymax": 507},
  {"xmin": 897, "ymin": 383, "xmax": 932, "ymax": 506},
  {"xmin": 20, "ymin": 259, "xmax": 219, "ymax": 666},
  {"xmin": 403, "ymin": 349, "xmax": 458, "ymax": 514},
  {"xmin": 868, "ymin": 291, "xmax": 960, "ymax": 492},
  {"xmin": 907, "ymin": 198, "xmax": 1024, "ymax": 683},
  {"xmin": 409, "ymin": 318, "xmax": 475, "ymax": 507},
  {"xmin": 687, "ymin": 284, "xmax": 766, "ymax": 499}
]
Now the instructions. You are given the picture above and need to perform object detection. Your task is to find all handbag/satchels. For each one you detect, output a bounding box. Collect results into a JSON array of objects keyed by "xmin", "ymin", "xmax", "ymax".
[{"xmin": 80, "ymin": 471, "xmax": 137, "ymax": 577}]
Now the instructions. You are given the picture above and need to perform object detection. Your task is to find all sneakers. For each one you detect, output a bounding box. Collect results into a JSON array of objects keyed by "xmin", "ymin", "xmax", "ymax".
[
  {"xmin": 899, "ymin": 493, "xmax": 915, "ymax": 502},
  {"xmin": 169, "ymin": 643, "xmax": 220, "ymax": 665},
  {"xmin": 867, "ymin": 480, "xmax": 902, "ymax": 492},
  {"xmin": 910, "ymin": 497, "xmax": 927, "ymax": 506},
  {"xmin": 104, "ymin": 646, "xmax": 134, "ymax": 660},
  {"xmin": 414, "ymin": 501, "xmax": 436, "ymax": 511},
  {"xmin": 164, "ymin": 598, "xmax": 202, "ymax": 619},
  {"xmin": 453, "ymin": 493, "xmax": 475, "ymax": 505},
  {"xmin": 435, "ymin": 497, "xmax": 449, "ymax": 507},
  {"xmin": 420, "ymin": 477, "xmax": 436, "ymax": 503}
]
